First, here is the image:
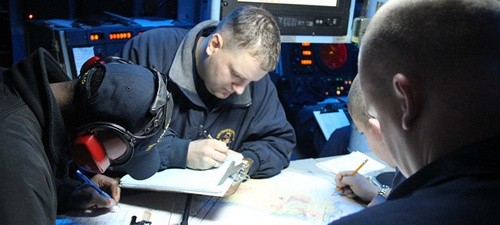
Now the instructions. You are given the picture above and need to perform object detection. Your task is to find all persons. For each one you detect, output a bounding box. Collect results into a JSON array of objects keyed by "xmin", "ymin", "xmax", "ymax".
[
  {"xmin": 332, "ymin": 74, "xmax": 414, "ymax": 209},
  {"xmin": 112, "ymin": 5, "xmax": 297, "ymax": 180},
  {"xmin": 0, "ymin": 43, "xmax": 172, "ymax": 225},
  {"xmin": 325, "ymin": 0, "xmax": 499, "ymax": 225}
]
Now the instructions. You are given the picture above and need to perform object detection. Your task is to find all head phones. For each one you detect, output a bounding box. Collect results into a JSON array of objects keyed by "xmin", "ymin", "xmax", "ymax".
[{"xmin": 64, "ymin": 55, "xmax": 170, "ymax": 174}]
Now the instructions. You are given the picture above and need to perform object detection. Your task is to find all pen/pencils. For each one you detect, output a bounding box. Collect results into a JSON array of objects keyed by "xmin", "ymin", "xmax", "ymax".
[
  {"xmin": 332, "ymin": 159, "xmax": 368, "ymax": 196},
  {"xmin": 203, "ymin": 131, "xmax": 213, "ymax": 140},
  {"xmin": 77, "ymin": 170, "xmax": 121, "ymax": 209}
]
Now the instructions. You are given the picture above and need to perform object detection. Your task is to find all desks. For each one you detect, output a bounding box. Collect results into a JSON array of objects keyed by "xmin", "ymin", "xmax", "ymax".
[{"xmin": 56, "ymin": 151, "xmax": 396, "ymax": 225}]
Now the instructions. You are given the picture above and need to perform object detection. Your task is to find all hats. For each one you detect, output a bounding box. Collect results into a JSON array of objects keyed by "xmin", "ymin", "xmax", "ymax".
[{"xmin": 76, "ymin": 61, "xmax": 164, "ymax": 180}]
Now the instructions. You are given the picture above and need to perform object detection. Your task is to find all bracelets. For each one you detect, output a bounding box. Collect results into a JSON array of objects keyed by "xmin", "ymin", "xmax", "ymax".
[{"xmin": 377, "ymin": 184, "xmax": 389, "ymax": 198}]
